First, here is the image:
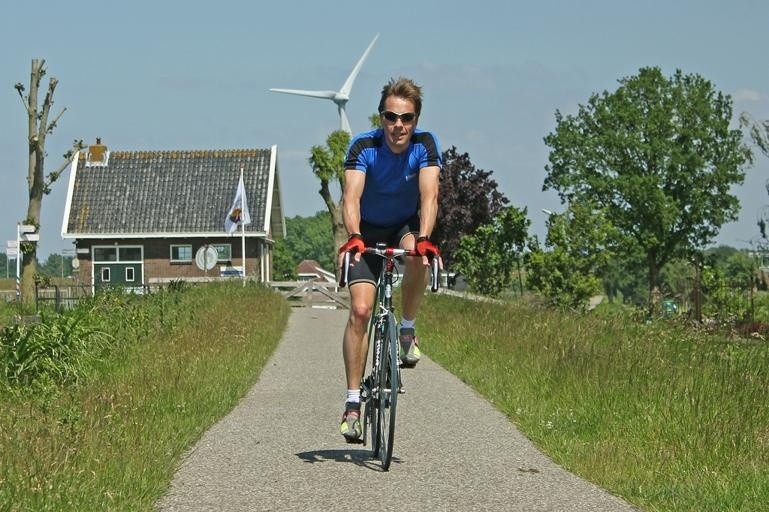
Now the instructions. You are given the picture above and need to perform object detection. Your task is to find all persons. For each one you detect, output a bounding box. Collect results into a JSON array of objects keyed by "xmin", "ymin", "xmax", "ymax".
[{"xmin": 339, "ymin": 76, "xmax": 443, "ymax": 443}]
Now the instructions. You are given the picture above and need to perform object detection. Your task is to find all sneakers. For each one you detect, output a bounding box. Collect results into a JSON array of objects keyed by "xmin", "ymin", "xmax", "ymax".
[
  {"xmin": 399, "ymin": 328, "xmax": 421, "ymax": 364},
  {"xmin": 340, "ymin": 402, "xmax": 362, "ymax": 439}
]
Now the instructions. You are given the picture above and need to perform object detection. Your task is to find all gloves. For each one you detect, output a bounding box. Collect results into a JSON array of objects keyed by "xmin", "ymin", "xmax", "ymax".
[
  {"xmin": 417, "ymin": 237, "xmax": 440, "ymax": 256},
  {"xmin": 337, "ymin": 234, "xmax": 366, "ymax": 254}
]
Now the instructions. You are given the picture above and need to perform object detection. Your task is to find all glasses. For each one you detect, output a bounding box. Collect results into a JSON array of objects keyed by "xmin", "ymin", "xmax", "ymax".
[{"xmin": 381, "ymin": 110, "xmax": 418, "ymax": 122}]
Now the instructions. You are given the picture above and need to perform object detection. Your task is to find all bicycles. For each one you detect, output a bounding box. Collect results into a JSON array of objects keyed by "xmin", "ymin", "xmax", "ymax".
[{"xmin": 342, "ymin": 241, "xmax": 439, "ymax": 473}]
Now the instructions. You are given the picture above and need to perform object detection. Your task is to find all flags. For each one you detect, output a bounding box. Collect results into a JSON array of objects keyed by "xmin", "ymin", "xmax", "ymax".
[{"xmin": 223, "ymin": 174, "xmax": 253, "ymax": 236}]
